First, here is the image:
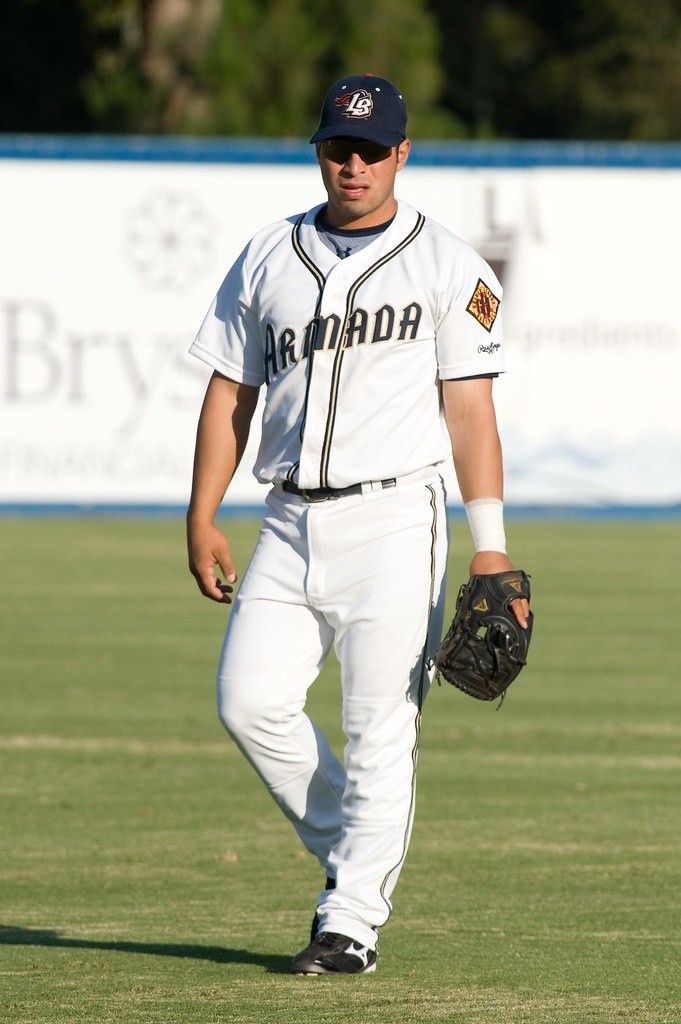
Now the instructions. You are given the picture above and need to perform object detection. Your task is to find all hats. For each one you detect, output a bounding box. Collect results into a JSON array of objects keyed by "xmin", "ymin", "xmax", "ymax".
[{"xmin": 310, "ymin": 74, "xmax": 407, "ymax": 148}]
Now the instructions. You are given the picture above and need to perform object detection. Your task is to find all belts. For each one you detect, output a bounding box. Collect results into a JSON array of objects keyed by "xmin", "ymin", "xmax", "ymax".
[{"xmin": 283, "ymin": 476, "xmax": 396, "ymax": 502}]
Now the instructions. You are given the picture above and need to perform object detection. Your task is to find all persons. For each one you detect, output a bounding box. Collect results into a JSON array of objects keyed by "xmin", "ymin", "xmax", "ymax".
[{"xmin": 186, "ymin": 70, "xmax": 530, "ymax": 977}]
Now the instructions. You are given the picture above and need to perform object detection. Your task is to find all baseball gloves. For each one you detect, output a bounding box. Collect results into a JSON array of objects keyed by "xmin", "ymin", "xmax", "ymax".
[{"xmin": 432, "ymin": 569, "xmax": 536, "ymax": 702}]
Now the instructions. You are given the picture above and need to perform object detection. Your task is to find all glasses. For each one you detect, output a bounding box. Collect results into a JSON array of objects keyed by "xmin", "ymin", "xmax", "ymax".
[{"xmin": 317, "ymin": 138, "xmax": 393, "ymax": 165}]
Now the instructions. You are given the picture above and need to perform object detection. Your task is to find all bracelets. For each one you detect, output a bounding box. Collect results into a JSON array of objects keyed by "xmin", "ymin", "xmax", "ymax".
[{"xmin": 466, "ymin": 496, "xmax": 507, "ymax": 555}]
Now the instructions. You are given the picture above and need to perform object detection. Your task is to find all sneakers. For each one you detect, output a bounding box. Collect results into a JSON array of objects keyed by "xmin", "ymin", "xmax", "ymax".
[{"xmin": 289, "ymin": 930, "xmax": 378, "ymax": 977}]
